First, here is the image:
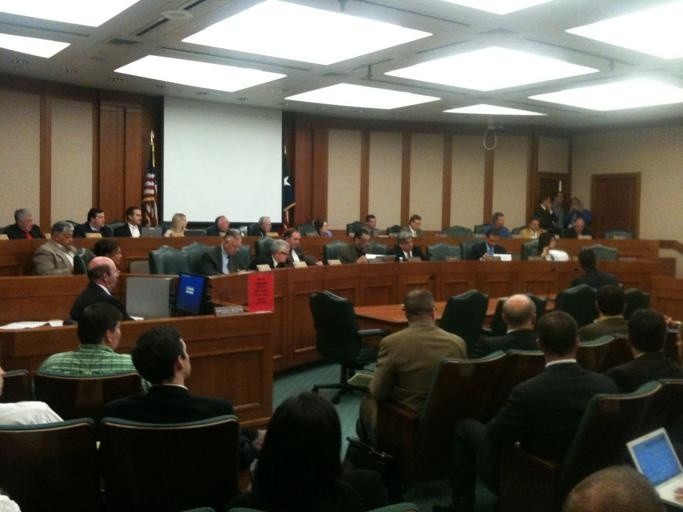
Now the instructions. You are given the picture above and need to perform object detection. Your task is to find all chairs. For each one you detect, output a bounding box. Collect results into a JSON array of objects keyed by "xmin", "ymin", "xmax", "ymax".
[{"xmin": 0, "ymin": 221, "xmax": 682, "ymax": 512}]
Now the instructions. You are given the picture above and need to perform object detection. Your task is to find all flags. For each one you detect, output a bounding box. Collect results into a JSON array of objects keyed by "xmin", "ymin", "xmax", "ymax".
[
  {"xmin": 281, "ymin": 152, "xmax": 296, "ymax": 232},
  {"xmin": 142, "ymin": 142, "xmax": 158, "ymax": 226}
]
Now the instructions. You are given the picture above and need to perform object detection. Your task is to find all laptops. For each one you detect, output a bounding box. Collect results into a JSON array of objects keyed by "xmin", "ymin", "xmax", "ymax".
[
  {"xmin": 140, "ymin": 227, "xmax": 162, "ymax": 237},
  {"xmin": 185, "ymin": 230, "xmax": 205, "ymax": 236},
  {"xmin": 625, "ymin": 428, "xmax": 683, "ymax": 508}
]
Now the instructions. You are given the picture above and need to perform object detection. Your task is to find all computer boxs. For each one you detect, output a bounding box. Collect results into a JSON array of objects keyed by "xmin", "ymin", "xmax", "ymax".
[{"xmin": 126, "ymin": 276, "xmax": 173, "ymax": 318}]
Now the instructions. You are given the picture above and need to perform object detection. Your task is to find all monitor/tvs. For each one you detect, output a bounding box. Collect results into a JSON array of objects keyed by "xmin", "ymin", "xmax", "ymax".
[{"xmin": 171, "ymin": 273, "xmax": 210, "ymax": 315}]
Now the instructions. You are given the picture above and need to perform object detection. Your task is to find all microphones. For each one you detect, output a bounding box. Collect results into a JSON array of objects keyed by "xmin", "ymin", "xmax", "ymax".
[
  {"xmin": 526, "ymin": 267, "xmax": 556, "ymax": 294},
  {"xmin": 402, "ymin": 274, "xmax": 435, "ymax": 310},
  {"xmin": 548, "ymin": 267, "xmax": 579, "ymax": 294}
]
